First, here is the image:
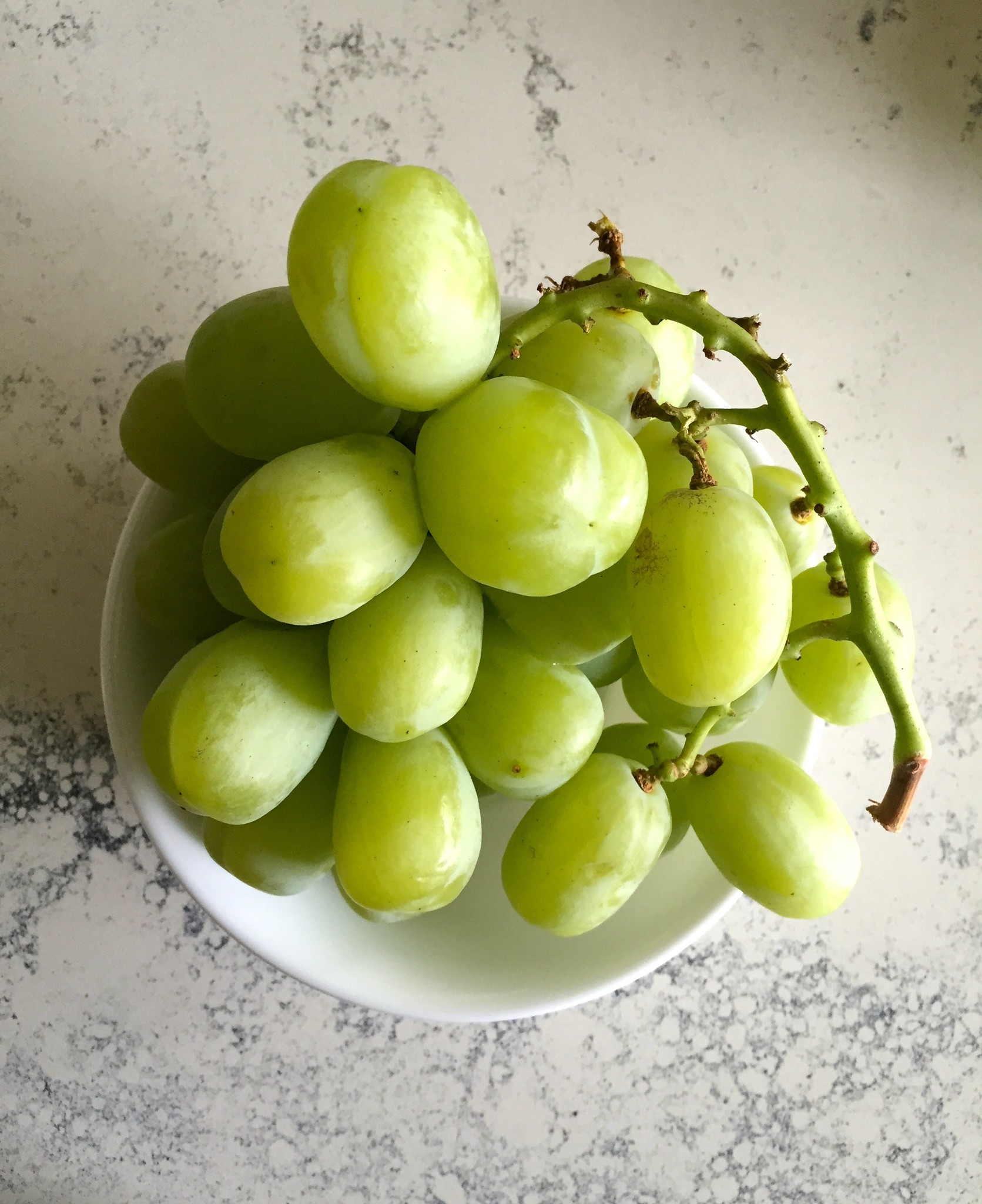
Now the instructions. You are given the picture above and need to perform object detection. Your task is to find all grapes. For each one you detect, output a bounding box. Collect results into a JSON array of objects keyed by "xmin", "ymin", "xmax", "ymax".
[{"xmin": 114, "ymin": 156, "xmax": 912, "ymax": 942}]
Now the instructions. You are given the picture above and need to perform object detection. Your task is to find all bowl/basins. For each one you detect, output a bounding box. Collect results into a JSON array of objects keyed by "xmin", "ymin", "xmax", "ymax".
[{"xmin": 95, "ymin": 293, "xmax": 826, "ymax": 1028}]
